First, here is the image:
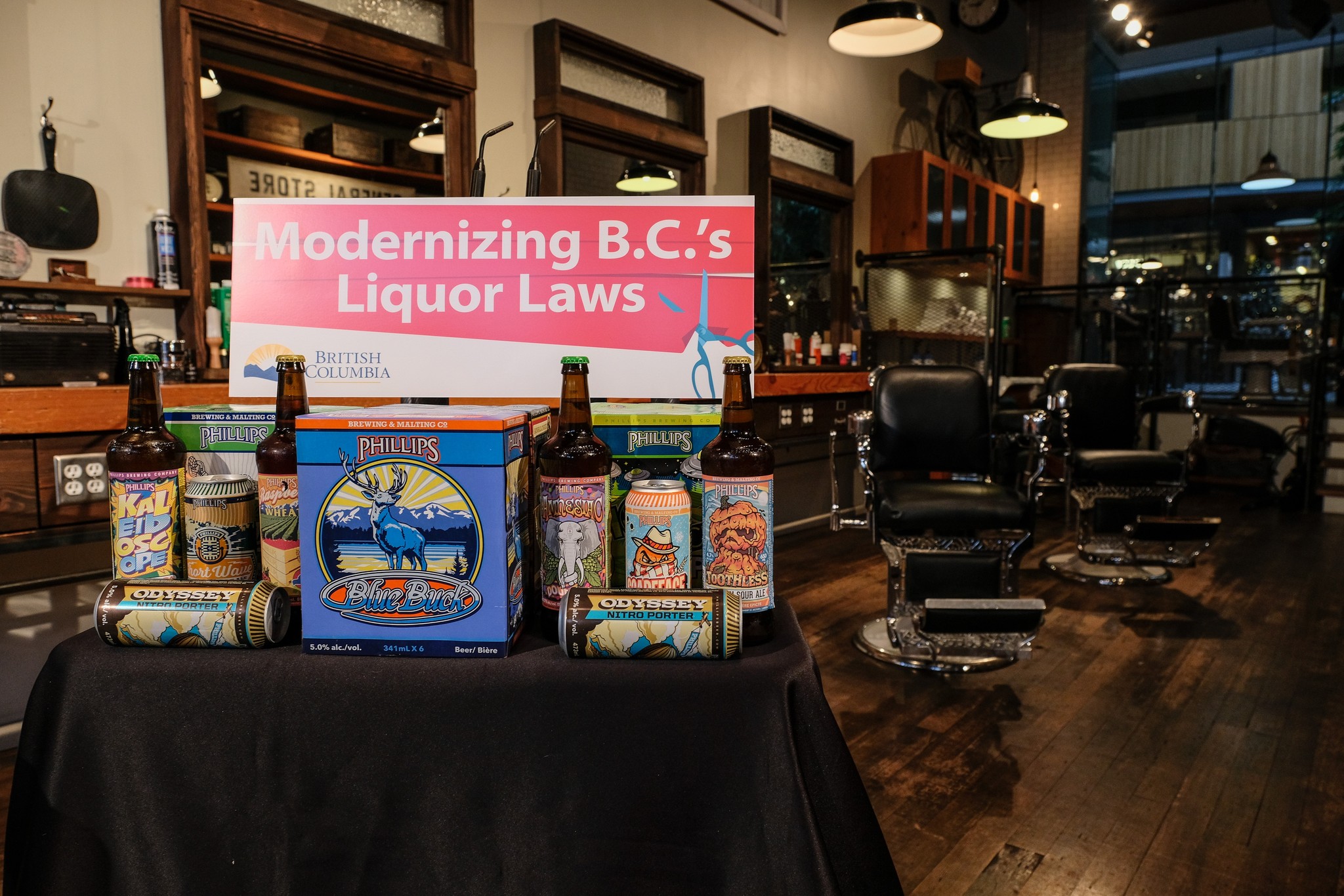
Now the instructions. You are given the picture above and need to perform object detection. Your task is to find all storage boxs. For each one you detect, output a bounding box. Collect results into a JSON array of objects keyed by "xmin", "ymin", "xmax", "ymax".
[
  {"xmin": 293, "ymin": 404, "xmax": 552, "ymax": 657},
  {"xmin": 164, "ymin": 405, "xmax": 365, "ymax": 571}
]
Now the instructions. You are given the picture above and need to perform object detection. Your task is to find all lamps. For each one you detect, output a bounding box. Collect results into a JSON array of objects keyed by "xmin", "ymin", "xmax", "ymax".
[
  {"xmin": 979, "ymin": 69, "xmax": 1069, "ymax": 140},
  {"xmin": 616, "ymin": 160, "xmax": 678, "ymax": 192},
  {"xmin": 200, "ymin": 64, "xmax": 222, "ymax": 99},
  {"xmin": 1241, "ymin": 150, "xmax": 1295, "ymax": 190},
  {"xmin": 409, "ymin": 108, "xmax": 445, "ymax": 154},
  {"xmin": 828, "ymin": 0, "xmax": 944, "ymax": 58}
]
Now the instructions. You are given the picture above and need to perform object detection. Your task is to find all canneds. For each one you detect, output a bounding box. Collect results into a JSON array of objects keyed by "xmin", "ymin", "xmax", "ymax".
[
  {"xmin": 625, "ymin": 480, "xmax": 692, "ymax": 590},
  {"xmin": 183, "ymin": 475, "xmax": 259, "ymax": 581},
  {"xmin": 94, "ymin": 577, "xmax": 290, "ymax": 649},
  {"xmin": 558, "ymin": 587, "xmax": 743, "ymax": 660}
]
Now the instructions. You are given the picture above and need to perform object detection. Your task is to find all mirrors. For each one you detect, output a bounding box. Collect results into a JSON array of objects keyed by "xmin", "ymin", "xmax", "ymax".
[
  {"xmin": 769, "ymin": 178, "xmax": 846, "ymax": 364},
  {"xmin": 561, "ymin": 123, "xmax": 699, "ymax": 197}
]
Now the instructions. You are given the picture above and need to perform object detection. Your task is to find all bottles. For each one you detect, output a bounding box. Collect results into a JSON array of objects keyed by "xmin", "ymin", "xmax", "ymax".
[
  {"xmin": 700, "ymin": 355, "xmax": 779, "ymax": 643},
  {"xmin": 209, "ymin": 279, "xmax": 232, "ymax": 353},
  {"xmin": 791, "ymin": 332, "xmax": 802, "ymax": 364},
  {"xmin": 539, "ymin": 356, "xmax": 612, "ymax": 634},
  {"xmin": 810, "ymin": 331, "xmax": 822, "ymax": 365},
  {"xmin": 105, "ymin": 353, "xmax": 188, "ymax": 583},
  {"xmin": 152, "ymin": 208, "xmax": 179, "ymax": 290},
  {"xmin": 256, "ymin": 354, "xmax": 311, "ymax": 643},
  {"xmin": 125, "ymin": 277, "xmax": 155, "ymax": 288}
]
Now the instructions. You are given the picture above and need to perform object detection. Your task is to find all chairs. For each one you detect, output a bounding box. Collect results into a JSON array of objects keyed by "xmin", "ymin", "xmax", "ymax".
[{"xmin": 828, "ymin": 364, "xmax": 1221, "ymax": 676}]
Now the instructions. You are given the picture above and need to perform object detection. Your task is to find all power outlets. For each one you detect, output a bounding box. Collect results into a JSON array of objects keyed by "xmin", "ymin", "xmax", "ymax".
[
  {"xmin": 779, "ymin": 405, "xmax": 793, "ymax": 429},
  {"xmin": 53, "ymin": 453, "xmax": 108, "ymax": 506},
  {"xmin": 800, "ymin": 405, "xmax": 814, "ymax": 428}
]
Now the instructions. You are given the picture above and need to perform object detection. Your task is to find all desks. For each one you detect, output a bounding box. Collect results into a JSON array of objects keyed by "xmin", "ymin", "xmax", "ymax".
[{"xmin": 0, "ymin": 598, "xmax": 904, "ymax": 896}]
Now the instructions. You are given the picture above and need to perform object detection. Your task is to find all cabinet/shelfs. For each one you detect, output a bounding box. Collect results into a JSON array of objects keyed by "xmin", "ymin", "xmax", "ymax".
[
  {"xmin": 871, "ymin": 151, "xmax": 1045, "ymax": 285},
  {"xmin": 200, "ymin": 59, "xmax": 444, "ymax": 263}
]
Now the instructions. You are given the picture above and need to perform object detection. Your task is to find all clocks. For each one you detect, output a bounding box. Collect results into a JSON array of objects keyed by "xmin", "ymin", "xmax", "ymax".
[{"xmin": 957, "ymin": 0, "xmax": 999, "ymax": 27}]
